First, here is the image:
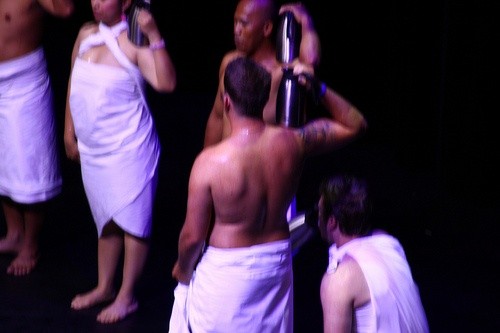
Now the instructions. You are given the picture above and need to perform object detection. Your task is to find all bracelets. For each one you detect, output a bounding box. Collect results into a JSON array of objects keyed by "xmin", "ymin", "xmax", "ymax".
[{"xmin": 148, "ymin": 38, "xmax": 165, "ymax": 49}]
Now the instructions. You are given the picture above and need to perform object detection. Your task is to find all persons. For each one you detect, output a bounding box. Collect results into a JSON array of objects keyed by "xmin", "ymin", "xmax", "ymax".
[
  {"xmin": 171, "ymin": 56, "xmax": 367, "ymax": 333},
  {"xmin": 0, "ymin": 0, "xmax": 75, "ymax": 276},
  {"xmin": 317, "ymin": 172, "xmax": 429, "ymax": 333},
  {"xmin": 203, "ymin": 0, "xmax": 320, "ymax": 261},
  {"xmin": 64, "ymin": 0, "xmax": 176, "ymax": 325}
]
